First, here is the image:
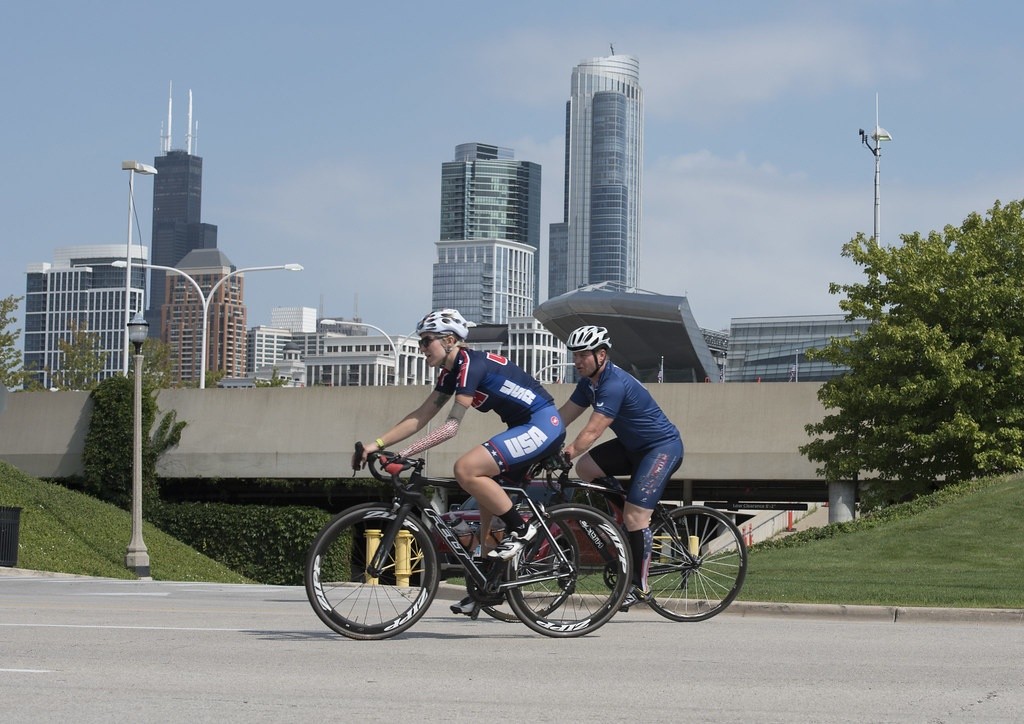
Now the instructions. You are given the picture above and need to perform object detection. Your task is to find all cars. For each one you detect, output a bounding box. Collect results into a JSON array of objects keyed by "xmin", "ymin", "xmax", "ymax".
[{"xmin": 435, "ymin": 479, "xmax": 628, "ymax": 577}]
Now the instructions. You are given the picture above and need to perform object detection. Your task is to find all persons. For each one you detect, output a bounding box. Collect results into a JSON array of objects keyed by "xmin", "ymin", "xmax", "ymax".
[
  {"xmin": 558, "ymin": 325, "xmax": 683, "ymax": 608},
  {"xmin": 351, "ymin": 309, "xmax": 567, "ymax": 613}
]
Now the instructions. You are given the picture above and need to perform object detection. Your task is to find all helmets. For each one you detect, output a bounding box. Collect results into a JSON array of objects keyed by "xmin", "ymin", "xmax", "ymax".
[
  {"xmin": 416, "ymin": 309, "xmax": 469, "ymax": 340},
  {"xmin": 565, "ymin": 326, "xmax": 613, "ymax": 352}
]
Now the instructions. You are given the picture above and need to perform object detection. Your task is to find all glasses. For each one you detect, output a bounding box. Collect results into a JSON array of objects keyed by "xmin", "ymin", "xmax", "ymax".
[{"xmin": 418, "ymin": 335, "xmax": 449, "ymax": 348}]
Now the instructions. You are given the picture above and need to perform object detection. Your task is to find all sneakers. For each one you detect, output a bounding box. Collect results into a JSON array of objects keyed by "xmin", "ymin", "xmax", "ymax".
[
  {"xmin": 450, "ymin": 596, "xmax": 476, "ymax": 613},
  {"xmin": 620, "ymin": 585, "xmax": 651, "ymax": 607},
  {"xmin": 487, "ymin": 525, "xmax": 537, "ymax": 562}
]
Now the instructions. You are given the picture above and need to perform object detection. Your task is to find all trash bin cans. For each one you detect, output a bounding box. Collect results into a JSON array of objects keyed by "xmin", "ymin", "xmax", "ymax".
[{"xmin": 0, "ymin": 505, "xmax": 24, "ymax": 567}]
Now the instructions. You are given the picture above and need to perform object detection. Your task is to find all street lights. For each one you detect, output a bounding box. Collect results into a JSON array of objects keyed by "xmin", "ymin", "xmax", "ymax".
[
  {"xmin": 120, "ymin": 160, "xmax": 158, "ymax": 379},
  {"xmin": 320, "ymin": 315, "xmax": 474, "ymax": 387},
  {"xmin": 111, "ymin": 260, "xmax": 304, "ymax": 388},
  {"xmin": 125, "ymin": 308, "xmax": 157, "ymax": 582},
  {"xmin": 858, "ymin": 91, "xmax": 893, "ymax": 331}
]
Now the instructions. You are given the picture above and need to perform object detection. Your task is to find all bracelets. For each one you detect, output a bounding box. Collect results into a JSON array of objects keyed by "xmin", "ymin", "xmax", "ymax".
[{"xmin": 375, "ymin": 438, "xmax": 384, "ymax": 451}]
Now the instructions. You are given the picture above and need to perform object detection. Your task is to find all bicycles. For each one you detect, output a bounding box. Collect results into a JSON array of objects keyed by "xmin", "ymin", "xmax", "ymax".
[
  {"xmin": 458, "ymin": 445, "xmax": 751, "ymax": 624},
  {"xmin": 305, "ymin": 446, "xmax": 634, "ymax": 641}
]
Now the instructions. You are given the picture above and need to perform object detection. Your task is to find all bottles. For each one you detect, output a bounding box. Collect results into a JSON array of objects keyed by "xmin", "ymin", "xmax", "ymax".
[
  {"xmin": 475, "ymin": 545, "xmax": 480, "ymax": 556},
  {"xmin": 485, "ymin": 516, "xmax": 506, "ymax": 548},
  {"xmin": 448, "ymin": 511, "xmax": 479, "ymax": 553}
]
